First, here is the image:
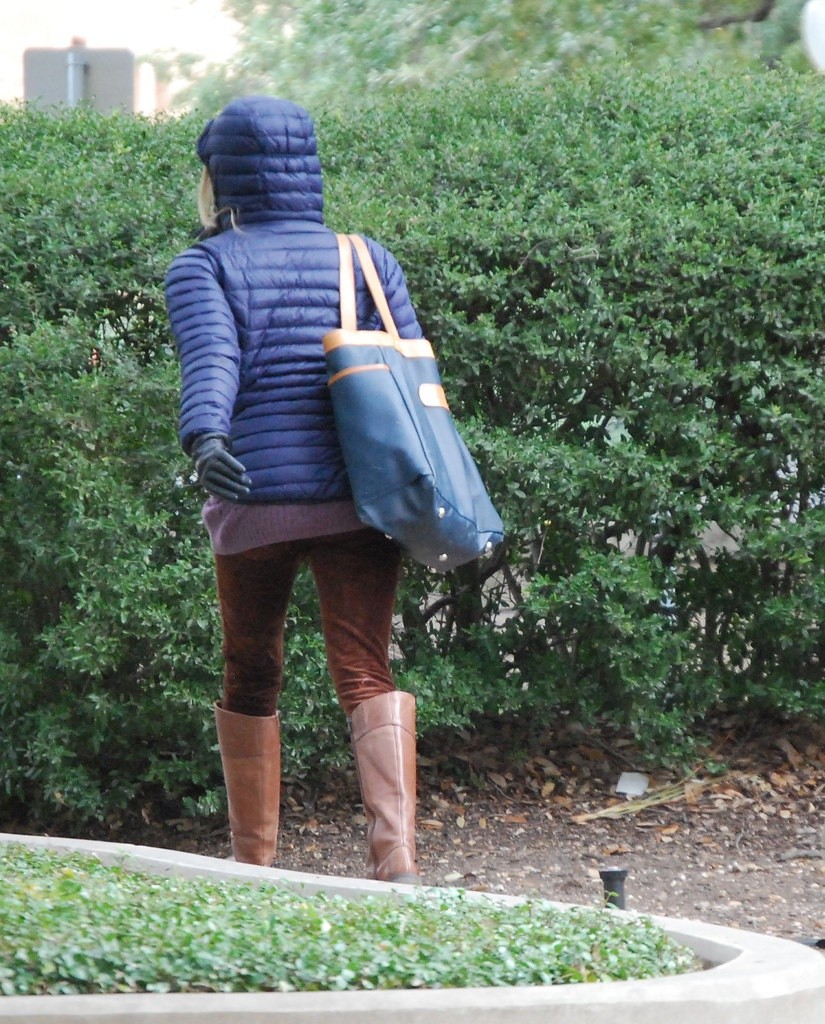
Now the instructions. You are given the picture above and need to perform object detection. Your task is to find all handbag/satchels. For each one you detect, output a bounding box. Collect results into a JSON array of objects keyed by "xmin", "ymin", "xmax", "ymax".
[{"xmin": 323, "ymin": 233, "xmax": 504, "ymax": 573}]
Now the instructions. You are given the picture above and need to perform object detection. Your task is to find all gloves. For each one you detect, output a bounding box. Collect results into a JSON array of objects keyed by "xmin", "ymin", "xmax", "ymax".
[{"xmin": 190, "ymin": 430, "xmax": 252, "ymax": 501}]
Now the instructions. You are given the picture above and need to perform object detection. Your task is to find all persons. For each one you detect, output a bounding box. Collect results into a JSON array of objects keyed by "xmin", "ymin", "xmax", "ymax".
[{"xmin": 163, "ymin": 97, "xmax": 422, "ymax": 885}]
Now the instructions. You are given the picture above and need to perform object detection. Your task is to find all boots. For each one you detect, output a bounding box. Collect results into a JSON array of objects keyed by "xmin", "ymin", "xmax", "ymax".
[
  {"xmin": 346, "ymin": 691, "xmax": 420, "ymax": 888},
  {"xmin": 214, "ymin": 701, "xmax": 281, "ymax": 867}
]
